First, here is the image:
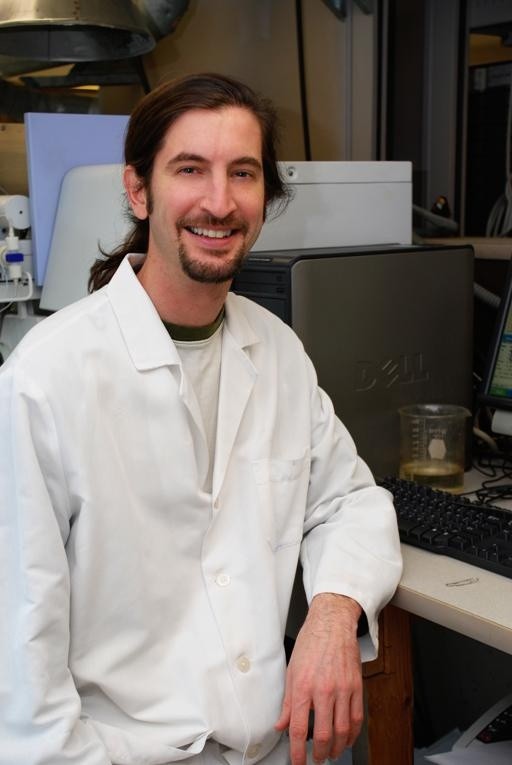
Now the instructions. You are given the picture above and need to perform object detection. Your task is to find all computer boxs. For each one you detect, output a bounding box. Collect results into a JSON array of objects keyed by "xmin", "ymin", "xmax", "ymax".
[{"xmin": 232, "ymin": 243, "xmax": 475, "ymax": 477}]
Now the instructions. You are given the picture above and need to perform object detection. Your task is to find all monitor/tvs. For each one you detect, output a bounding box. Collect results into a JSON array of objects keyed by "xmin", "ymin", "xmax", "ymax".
[{"xmin": 480, "ymin": 273, "xmax": 512, "ymax": 407}]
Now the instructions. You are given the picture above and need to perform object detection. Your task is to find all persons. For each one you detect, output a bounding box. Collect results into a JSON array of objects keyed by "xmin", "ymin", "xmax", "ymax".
[{"xmin": 0, "ymin": 67, "xmax": 407, "ymax": 763}]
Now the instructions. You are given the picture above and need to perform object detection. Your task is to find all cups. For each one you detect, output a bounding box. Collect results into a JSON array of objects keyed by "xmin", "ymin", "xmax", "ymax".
[{"xmin": 395, "ymin": 399, "xmax": 472, "ymax": 499}]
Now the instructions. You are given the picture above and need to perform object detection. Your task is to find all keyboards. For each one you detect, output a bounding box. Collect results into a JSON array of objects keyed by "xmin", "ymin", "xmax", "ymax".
[{"xmin": 376, "ymin": 474, "xmax": 512, "ymax": 579}]
